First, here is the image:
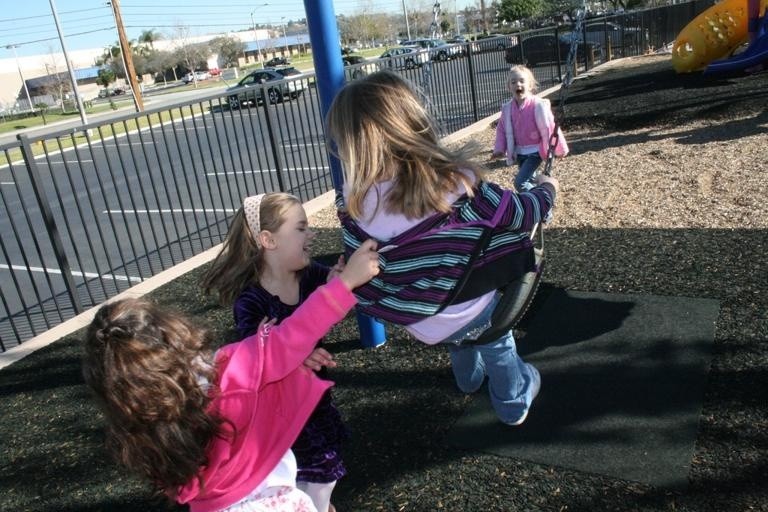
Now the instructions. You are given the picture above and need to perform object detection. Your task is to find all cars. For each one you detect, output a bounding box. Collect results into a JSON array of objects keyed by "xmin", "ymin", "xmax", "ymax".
[{"xmin": 98, "ymin": 56, "xmax": 291, "ymax": 99}]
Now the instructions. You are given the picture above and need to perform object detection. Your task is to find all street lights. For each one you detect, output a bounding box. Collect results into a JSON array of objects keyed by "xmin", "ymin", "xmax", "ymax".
[
  {"xmin": 6, "ymin": 44, "xmax": 34, "ymax": 113},
  {"xmin": 249, "ymin": 0, "xmax": 369, "ymax": 69}
]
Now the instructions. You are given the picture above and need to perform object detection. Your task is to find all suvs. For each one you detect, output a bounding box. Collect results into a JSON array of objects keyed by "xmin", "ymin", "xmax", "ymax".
[{"xmin": 225, "ymin": 65, "xmax": 308, "ymax": 108}]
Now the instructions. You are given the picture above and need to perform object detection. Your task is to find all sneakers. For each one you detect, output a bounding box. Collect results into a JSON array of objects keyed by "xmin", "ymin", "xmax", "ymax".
[
  {"xmin": 542, "ymin": 207, "xmax": 552, "ymax": 230},
  {"xmin": 503, "ymin": 362, "xmax": 542, "ymax": 425}
]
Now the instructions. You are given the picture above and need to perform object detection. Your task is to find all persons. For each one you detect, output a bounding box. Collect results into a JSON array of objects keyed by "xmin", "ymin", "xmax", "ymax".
[
  {"xmin": 325, "ymin": 71, "xmax": 560, "ymax": 427},
  {"xmin": 490, "ymin": 65, "xmax": 570, "ymax": 230},
  {"xmin": 85, "ymin": 238, "xmax": 380, "ymax": 512},
  {"xmin": 198, "ymin": 192, "xmax": 352, "ymax": 512}
]
